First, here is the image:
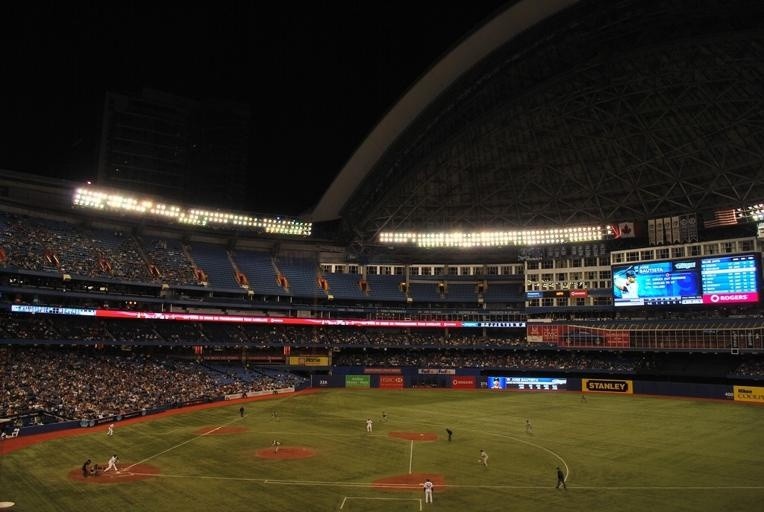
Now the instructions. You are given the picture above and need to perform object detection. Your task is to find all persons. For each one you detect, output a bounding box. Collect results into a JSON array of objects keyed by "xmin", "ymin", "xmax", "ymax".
[
  {"xmin": 1, "ymin": 294, "xmax": 648, "ymax": 440},
  {"xmin": 423, "ymin": 479, "xmax": 433, "ymax": 504},
  {"xmin": 481, "ymin": 449, "xmax": 488, "ymax": 467},
  {"xmin": 82, "ymin": 454, "xmax": 119, "ymax": 477},
  {"xmin": 709, "ymin": 304, "xmax": 764, "ymax": 378},
  {"xmin": 614, "ymin": 269, "xmax": 640, "ymax": 300},
  {"xmin": 1, "ymin": 214, "xmax": 208, "ymax": 290},
  {"xmin": 556, "ymin": 467, "xmax": 566, "ymax": 489}
]
